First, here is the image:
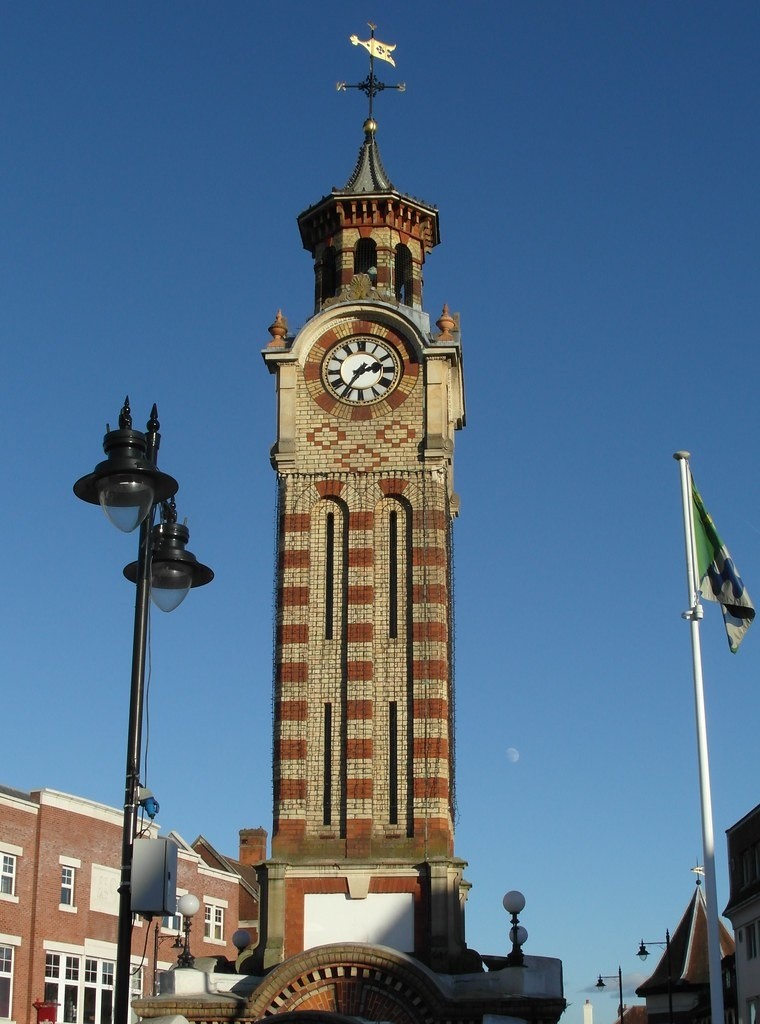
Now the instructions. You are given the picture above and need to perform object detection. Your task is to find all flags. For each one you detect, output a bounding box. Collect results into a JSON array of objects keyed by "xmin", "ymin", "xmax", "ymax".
[{"xmin": 687, "ymin": 467, "xmax": 756, "ymax": 653}]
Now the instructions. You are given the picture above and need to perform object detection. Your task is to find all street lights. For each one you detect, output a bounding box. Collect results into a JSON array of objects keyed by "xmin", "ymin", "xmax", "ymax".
[
  {"xmin": 635, "ymin": 928, "xmax": 674, "ymax": 1024},
  {"xmin": 594, "ymin": 965, "xmax": 624, "ymax": 1024},
  {"xmin": 71, "ymin": 394, "xmax": 216, "ymax": 1024}
]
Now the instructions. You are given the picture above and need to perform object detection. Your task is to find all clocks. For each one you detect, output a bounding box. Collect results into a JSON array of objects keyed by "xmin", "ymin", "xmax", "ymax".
[{"xmin": 321, "ymin": 338, "xmax": 400, "ymax": 406}]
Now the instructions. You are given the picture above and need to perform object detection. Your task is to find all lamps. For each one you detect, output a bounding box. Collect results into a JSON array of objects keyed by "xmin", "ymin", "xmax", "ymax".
[
  {"xmin": 503, "ymin": 890, "xmax": 527, "ymax": 913},
  {"xmin": 232, "ymin": 930, "xmax": 250, "ymax": 948},
  {"xmin": 178, "ymin": 894, "xmax": 199, "ymax": 915},
  {"xmin": 509, "ymin": 926, "xmax": 529, "ymax": 943}
]
